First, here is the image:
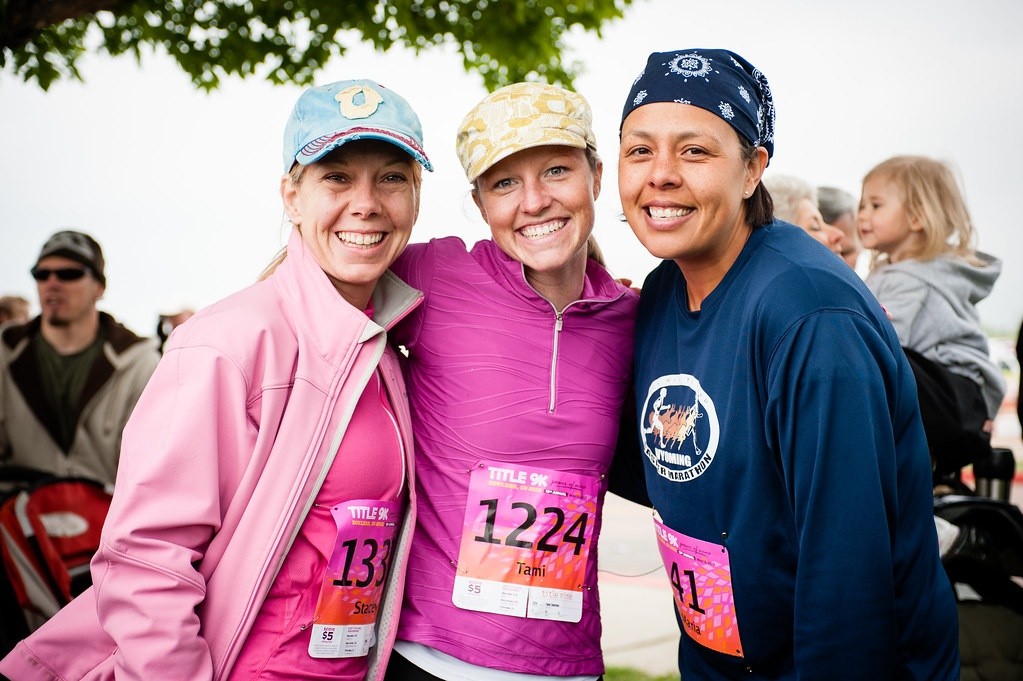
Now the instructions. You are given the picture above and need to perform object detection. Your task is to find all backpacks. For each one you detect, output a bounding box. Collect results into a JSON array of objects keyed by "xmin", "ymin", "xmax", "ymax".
[{"xmin": 0, "ymin": 464, "xmax": 114, "ymax": 634}]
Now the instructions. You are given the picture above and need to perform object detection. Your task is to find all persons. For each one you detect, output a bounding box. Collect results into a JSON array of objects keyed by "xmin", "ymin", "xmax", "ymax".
[
  {"xmin": 856, "ymin": 157, "xmax": 1008, "ymax": 492},
  {"xmin": 387, "ymin": 82, "xmax": 642, "ymax": 681},
  {"xmin": 765, "ymin": 175, "xmax": 863, "ymax": 271},
  {"xmin": 0, "ymin": 230, "xmax": 193, "ymax": 662},
  {"xmin": 619, "ymin": 49, "xmax": 962, "ymax": 681},
  {"xmin": 0, "ymin": 79, "xmax": 433, "ymax": 681}
]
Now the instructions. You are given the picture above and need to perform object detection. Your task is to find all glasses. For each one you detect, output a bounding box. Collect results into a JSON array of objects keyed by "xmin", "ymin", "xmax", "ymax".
[{"xmin": 34, "ymin": 269, "xmax": 85, "ymax": 281}]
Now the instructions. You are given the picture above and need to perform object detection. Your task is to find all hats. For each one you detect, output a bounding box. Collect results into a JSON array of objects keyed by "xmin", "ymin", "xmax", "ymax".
[
  {"xmin": 31, "ymin": 231, "xmax": 106, "ymax": 285},
  {"xmin": 283, "ymin": 79, "xmax": 434, "ymax": 177},
  {"xmin": 455, "ymin": 83, "xmax": 596, "ymax": 188}
]
